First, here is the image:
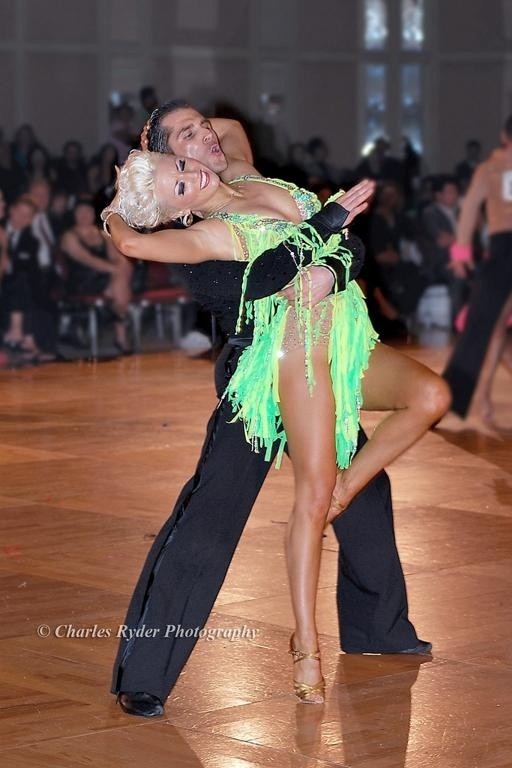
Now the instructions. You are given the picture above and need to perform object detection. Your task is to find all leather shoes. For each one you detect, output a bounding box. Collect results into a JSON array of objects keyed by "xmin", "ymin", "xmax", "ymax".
[
  {"xmin": 342, "ymin": 638, "xmax": 434, "ymax": 656},
  {"xmin": 118, "ymin": 691, "xmax": 166, "ymax": 716}
]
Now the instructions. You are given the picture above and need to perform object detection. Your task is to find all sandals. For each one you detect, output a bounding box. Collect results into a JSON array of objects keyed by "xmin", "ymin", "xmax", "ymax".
[{"xmin": 289, "ymin": 632, "xmax": 328, "ymax": 704}]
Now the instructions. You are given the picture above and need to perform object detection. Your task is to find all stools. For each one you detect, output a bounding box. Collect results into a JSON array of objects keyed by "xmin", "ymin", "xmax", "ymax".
[{"xmin": 75, "ymin": 283, "xmax": 220, "ymax": 361}]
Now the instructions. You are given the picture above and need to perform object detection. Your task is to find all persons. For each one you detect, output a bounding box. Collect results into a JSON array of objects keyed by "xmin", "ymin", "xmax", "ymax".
[
  {"xmin": 437, "ymin": 113, "xmax": 511, "ymax": 417},
  {"xmin": 104, "ymin": 97, "xmax": 437, "ymax": 719},
  {"xmin": 96, "ymin": 146, "xmax": 460, "ymax": 707},
  {"xmin": 448, "ymin": 155, "xmax": 511, "ymax": 421},
  {"xmin": 1, "ymin": 84, "xmax": 512, "ymax": 366}
]
